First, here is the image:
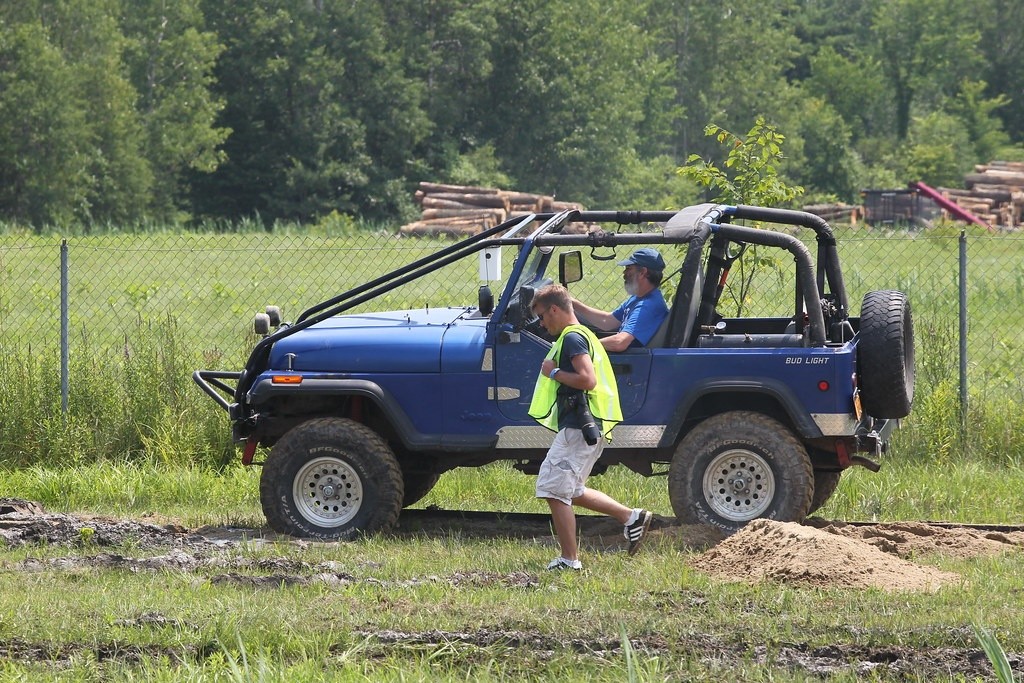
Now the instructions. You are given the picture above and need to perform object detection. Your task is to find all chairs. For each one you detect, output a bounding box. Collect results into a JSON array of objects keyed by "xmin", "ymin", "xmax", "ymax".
[{"xmin": 642, "ymin": 259, "xmax": 705, "ymax": 348}]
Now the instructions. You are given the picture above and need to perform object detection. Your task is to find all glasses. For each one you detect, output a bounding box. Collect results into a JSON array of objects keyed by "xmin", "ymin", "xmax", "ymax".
[{"xmin": 537, "ymin": 305, "xmax": 551, "ymax": 320}]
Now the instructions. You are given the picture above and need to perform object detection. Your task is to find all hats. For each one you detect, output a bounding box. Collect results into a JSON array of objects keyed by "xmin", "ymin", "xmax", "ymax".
[{"xmin": 618, "ymin": 248, "xmax": 666, "ymax": 272}]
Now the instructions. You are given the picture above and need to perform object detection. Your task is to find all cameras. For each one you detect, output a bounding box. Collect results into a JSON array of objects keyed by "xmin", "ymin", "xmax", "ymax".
[{"xmin": 558, "ymin": 383, "xmax": 602, "ymax": 446}]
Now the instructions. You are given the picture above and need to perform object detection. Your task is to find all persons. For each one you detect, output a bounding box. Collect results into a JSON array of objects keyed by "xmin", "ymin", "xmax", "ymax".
[
  {"xmin": 570, "ymin": 248, "xmax": 670, "ymax": 354},
  {"xmin": 529, "ymin": 283, "xmax": 655, "ymax": 572}
]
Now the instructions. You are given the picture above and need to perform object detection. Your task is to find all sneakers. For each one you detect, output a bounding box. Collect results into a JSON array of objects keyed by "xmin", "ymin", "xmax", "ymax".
[
  {"xmin": 627, "ymin": 508, "xmax": 652, "ymax": 557},
  {"xmin": 544, "ymin": 561, "xmax": 582, "ymax": 573}
]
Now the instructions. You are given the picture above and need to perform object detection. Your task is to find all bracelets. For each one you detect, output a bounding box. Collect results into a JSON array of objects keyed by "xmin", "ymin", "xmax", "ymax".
[{"xmin": 550, "ymin": 366, "xmax": 561, "ymax": 381}]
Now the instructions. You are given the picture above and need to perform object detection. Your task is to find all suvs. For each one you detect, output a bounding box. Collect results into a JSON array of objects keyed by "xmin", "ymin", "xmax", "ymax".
[{"xmin": 189, "ymin": 204, "xmax": 918, "ymax": 542}]
